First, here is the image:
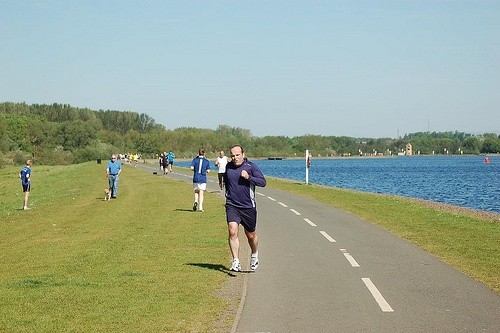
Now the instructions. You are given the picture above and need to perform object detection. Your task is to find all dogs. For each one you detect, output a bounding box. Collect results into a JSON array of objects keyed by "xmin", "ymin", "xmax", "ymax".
[{"xmin": 103, "ymin": 187, "xmax": 111, "ymax": 202}]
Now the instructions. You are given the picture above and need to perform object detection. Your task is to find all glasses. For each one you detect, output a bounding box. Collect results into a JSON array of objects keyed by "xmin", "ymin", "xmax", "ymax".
[{"xmin": 112, "ymin": 158, "xmax": 115, "ymax": 159}]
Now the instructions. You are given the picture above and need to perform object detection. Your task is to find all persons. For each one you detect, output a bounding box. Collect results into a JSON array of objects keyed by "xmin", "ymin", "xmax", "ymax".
[
  {"xmin": 115, "ymin": 152, "xmax": 143, "ymax": 168},
  {"xmin": 158, "ymin": 152, "xmax": 164, "ymax": 172},
  {"xmin": 105, "ymin": 153, "xmax": 122, "ymax": 198},
  {"xmin": 223, "ymin": 144, "xmax": 266, "ymax": 272},
  {"xmin": 190, "ymin": 148, "xmax": 211, "ymax": 212},
  {"xmin": 215, "ymin": 150, "xmax": 230, "ymax": 191},
  {"xmin": 162, "ymin": 151, "xmax": 169, "ymax": 175},
  {"xmin": 18, "ymin": 159, "xmax": 32, "ymax": 210},
  {"xmin": 155, "ymin": 153, "xmax": 159, "ymax": 159},
  {"xmin": 166, "ymin": 150, "xmax": 175, "ymax": 172}
]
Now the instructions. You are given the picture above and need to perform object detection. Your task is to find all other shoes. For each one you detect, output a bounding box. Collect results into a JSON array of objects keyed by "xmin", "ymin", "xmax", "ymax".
[
  {"xmin": 220, "ymin": 188, "xmax": 222, "ymax": 190},
  {"xmin": 113, "ymin": 195, "xmax": 116, "ymax": 198},
  {"xmin": 23, "ymin": 207, "xmax": 31, "ymax": 210},
  {"xmin": 193, "ymin": 202, "xmax": 198, "ymax": 211},
  {"xmin": 198, "ymin": 208, "xmax": 204, "ymax": 212}
]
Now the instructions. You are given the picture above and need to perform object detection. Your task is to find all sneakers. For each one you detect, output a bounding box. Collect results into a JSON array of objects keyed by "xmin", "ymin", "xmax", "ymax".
[
  {"xmin": 230, "ymin": 259, "xmax": 241, "ymax": 272},
  {"xmin": 250, "ymin": 250, "xmax": 259, "ymax": 270}
]
